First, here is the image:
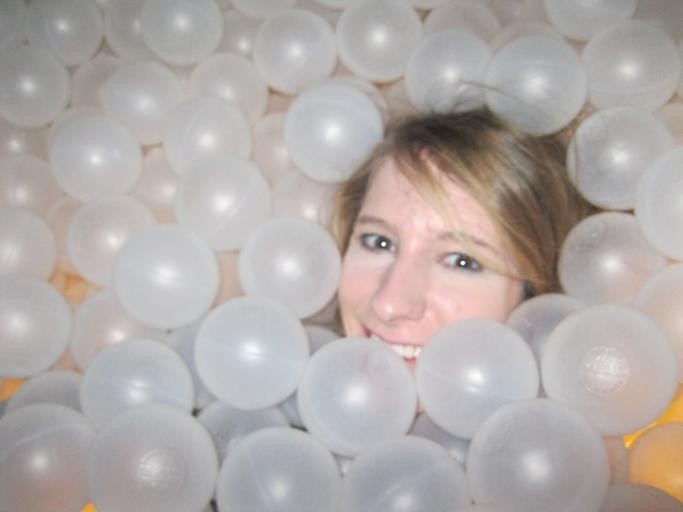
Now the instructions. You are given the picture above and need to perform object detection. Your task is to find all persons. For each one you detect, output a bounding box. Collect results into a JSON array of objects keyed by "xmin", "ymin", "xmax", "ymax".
[{"xmin": 335, "ymin": 107, "xmax": 600, "ymax": 410}]
